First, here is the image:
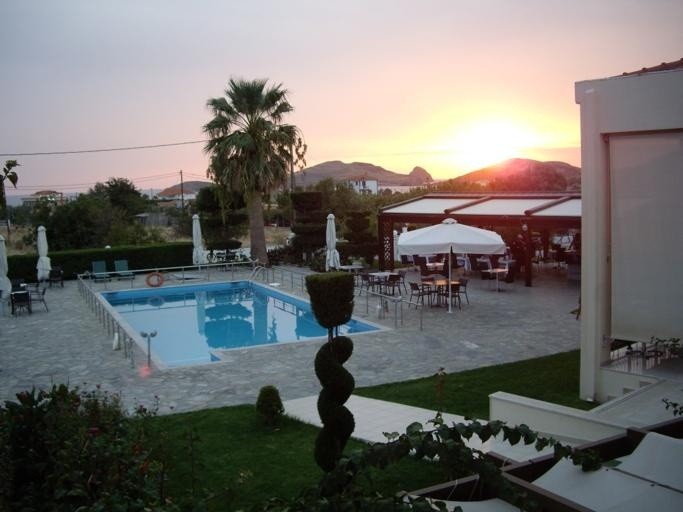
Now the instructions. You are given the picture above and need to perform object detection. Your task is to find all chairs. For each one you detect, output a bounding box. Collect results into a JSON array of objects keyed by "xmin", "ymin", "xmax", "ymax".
[
  {"xmin": 9, "ymin": 290, "xmax": 30, "ymax": 314},
  {"xmin": 27, "ymin": 287, "xmax": 51, "ymax": 312},
  {"xmin": 87, "ymin": 259, "xmax": 111, "ymax": 281},
  {"xmin": 24, "ymin": 279, "xmax": 40, "ymax": 294},
  {"xmin": 46, "ymin": 266, "xmax": 64, "ymax": 291},
  {"xmin": 110, "ymin": 260, "xmax": 139, "ymax": 282},
  {"xmin": 226, "ymin": 250, "xmax": 239, "ymax": 270},
  {"xmin": 205, "ymin": 252, "xmax": 221, "ymax": 270},
  {"xmin": 216, "ymin": 252, "xmax": 232, "ymax": 270},
  {"xmin": 331, "ymin": 253, "xmax": 520, "ymax": 314}
]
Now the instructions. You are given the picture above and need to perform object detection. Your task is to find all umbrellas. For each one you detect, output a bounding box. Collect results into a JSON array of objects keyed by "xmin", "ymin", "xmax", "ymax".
[
  {"xmin": 35, "ymin": 225, "xmax": 52, "ymax": 288},
  {"xmin": 192, "ymin": 213, "xmax": 205, "ymax": 272},
  {"xmin": 397, "ymin": 217, "xmax": 506, "ymax": 314},
  {"xmin": 325, "ymin": 213, "xmax": 340, "ymax": 272},
  {"xmin": 0, "ymin": 234, "xmax": 12, "ymax": 319}
]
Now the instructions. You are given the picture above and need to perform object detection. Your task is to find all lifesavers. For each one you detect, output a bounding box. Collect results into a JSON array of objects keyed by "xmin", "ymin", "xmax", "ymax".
[{"xmin": 146, "ymin": 272, "xmax": 163, "ymax": 287}]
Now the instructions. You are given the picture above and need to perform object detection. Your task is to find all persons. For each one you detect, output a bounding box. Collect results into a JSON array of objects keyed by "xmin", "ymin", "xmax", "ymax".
[{"xmin": 475, "ymin": 221, "xmax": 580, "ymax": 284}]
